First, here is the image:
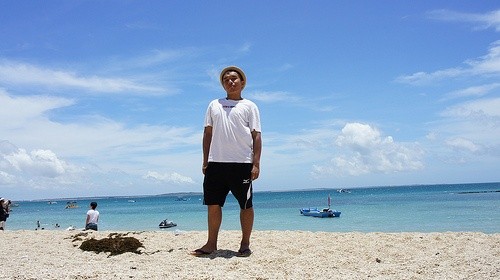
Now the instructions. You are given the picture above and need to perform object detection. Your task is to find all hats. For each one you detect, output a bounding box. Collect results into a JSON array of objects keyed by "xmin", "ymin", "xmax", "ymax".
[{"xmin": 219, "ymin": 66, "xmax": 246, "ymax": 90}]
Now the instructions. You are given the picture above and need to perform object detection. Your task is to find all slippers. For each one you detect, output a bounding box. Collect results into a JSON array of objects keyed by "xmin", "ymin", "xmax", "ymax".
[
  {"xmin": 237, "ymin": 249, "xmax": 251, "ymax": 257},
  {"xmin": 190, "ymin": 249, "xmax": 217, "ymax": 257}
]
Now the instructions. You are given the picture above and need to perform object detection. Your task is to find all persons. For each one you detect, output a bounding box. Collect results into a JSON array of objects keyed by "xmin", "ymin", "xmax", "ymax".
[
  {"xmin": 189, "ymin": 66, "xmax": 262, "ymax": 257},
  {"xmin": 0, "ymin": 196, "xmax": 9, "ymax": 231},
  {"xmin": 85, "ymin": 201, "xmax": 100, "ymax": 231}
]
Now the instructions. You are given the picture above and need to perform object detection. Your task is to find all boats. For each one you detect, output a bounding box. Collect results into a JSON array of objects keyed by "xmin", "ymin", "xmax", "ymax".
[
  {"xmin": 300, "ymin": 208, "xmax": 320, "ymax": 216},
  {"xmin": 158, "ymin": 219, "xmax": 177, "ymax": 228},
  {"xmin": 312, "ymin": 208, "xmax": 342, "ymax": 218}
]
